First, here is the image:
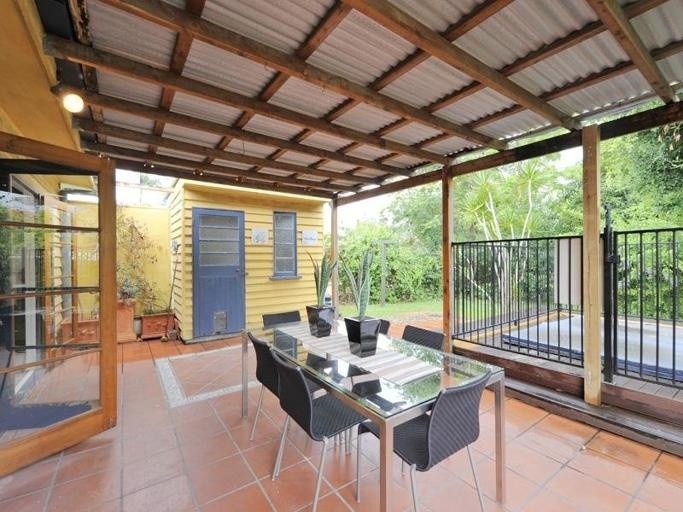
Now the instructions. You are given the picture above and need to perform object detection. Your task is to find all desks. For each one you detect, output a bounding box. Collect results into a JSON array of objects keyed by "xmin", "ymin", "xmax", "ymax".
[{"xmin": 242, "ymin": 322, "xmax": 503, "ymax": 511}]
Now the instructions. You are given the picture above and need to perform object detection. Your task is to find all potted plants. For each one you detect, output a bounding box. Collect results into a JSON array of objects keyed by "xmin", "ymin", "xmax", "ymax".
[
  {"xmin": 301, "ymin": 246, "xmax": 343, "ymax": 337},
  {"xmin": 139, "ymin": 307, "xmax": 175, "ymax": 341},
  {"xmin": 337, "ymin": 245, "xmax": 382, "ymax": 358}
]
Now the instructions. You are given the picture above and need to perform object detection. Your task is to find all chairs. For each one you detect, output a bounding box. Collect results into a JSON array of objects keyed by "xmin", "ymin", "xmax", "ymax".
[
  {"xmin": 270, "ymin": 351, "xmax": 372, "ymax": 511},
  {"xmin": 353, "ymin": 371, "xmax": 494, "ymax": 509},
  {"xmin": 261, "ymin": 309, "xmax": 302, "ymax": 326},
  {"xmin": 246, "ymin": 332, "xmax": 335, "ymax": 473},
  {"xmin": 375, "ymin": 317, "xmax": 391, "ymax": 332},
  {"xmin": 401, "ymin": 325, "xmax": 447, "ymax": 350}
]
